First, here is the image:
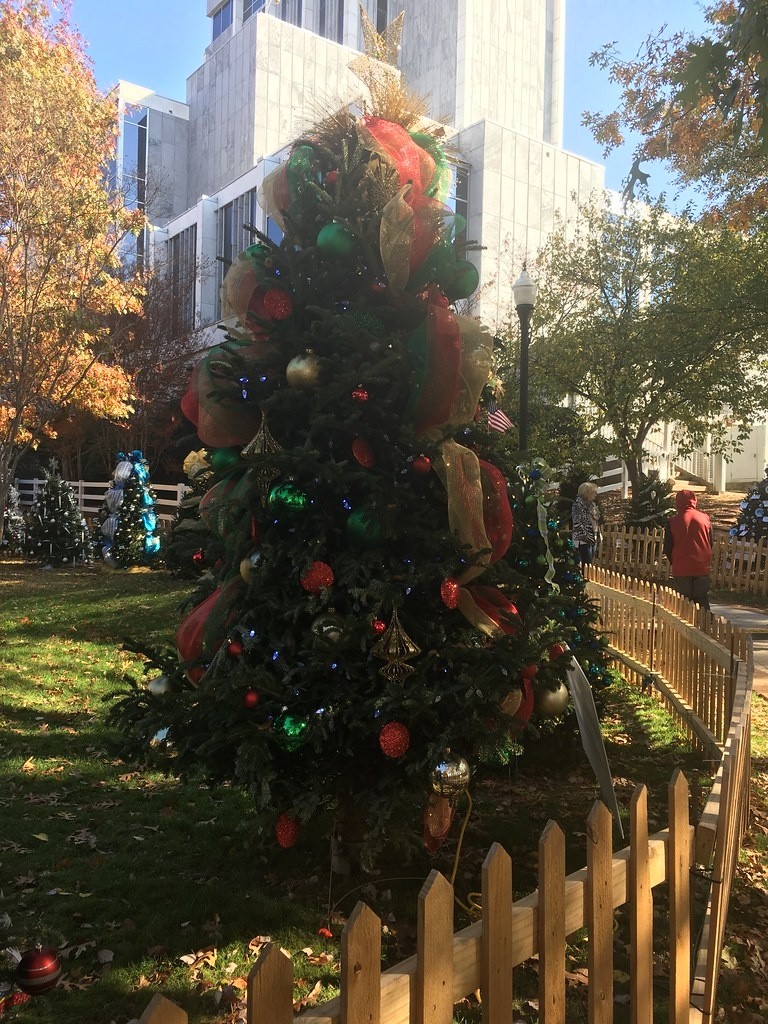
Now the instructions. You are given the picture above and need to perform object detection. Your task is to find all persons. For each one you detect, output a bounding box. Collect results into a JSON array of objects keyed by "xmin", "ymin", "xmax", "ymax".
[
  {"xmin": 664, "ymin": 490, "xmax": 713, "ymax": 623},
  {"xmin": 572, "ymin": 482, "xmax": 600, "ymax": 575}
]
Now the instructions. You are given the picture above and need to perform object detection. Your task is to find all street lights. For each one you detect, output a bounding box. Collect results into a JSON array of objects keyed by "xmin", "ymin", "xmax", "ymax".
[{"xmin": 510, "ymin": 259, "xmax": 538, "ymax": 460}]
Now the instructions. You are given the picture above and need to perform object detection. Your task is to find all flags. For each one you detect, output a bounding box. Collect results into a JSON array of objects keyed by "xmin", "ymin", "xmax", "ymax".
[{"xmin": 488, "ymin": 401, "xmax": 514, "ymax": 433}]
